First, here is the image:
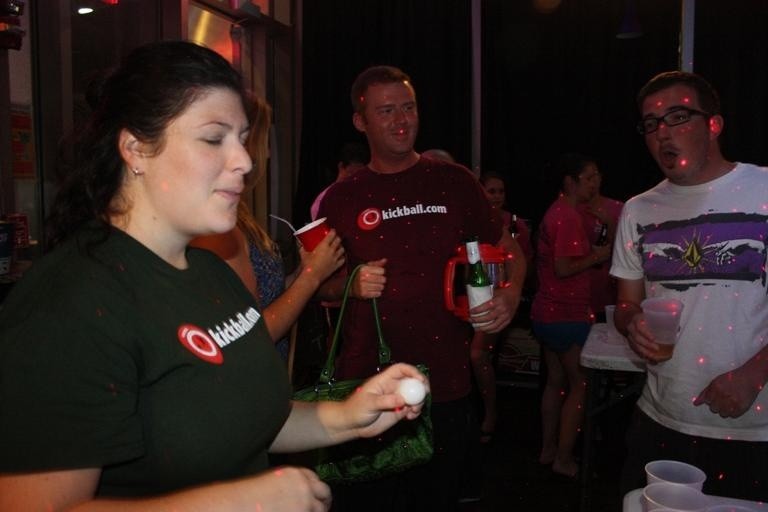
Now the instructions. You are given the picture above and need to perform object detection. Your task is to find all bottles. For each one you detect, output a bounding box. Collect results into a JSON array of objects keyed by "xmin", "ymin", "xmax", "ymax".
[
  {"xmin": 592, "ymin": 223, "xmax": 610, "ymax": 269},
  {"xmin": 508, "ymin": 214, "xmax": 518, "ymax": 239},
  {"xmin": 462, "ymin": 234, "xmax": 495, "ymax": 332}
]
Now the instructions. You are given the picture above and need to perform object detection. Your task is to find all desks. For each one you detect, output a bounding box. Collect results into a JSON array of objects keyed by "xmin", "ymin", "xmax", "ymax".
[{"xmin": 570, "ymin": 320, "xmax": 648, "ymax": 505}]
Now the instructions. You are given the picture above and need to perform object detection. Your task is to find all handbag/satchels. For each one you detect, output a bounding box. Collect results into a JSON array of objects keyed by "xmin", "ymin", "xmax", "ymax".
[{"xmin": 295, "ymin": 364, "xmax": 434, "ymax": 482}]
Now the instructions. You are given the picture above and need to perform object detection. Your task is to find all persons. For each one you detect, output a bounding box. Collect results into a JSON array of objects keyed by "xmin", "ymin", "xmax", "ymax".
[
  {"xmin": 188, "ymin": 89, "xmax": 344, "ymax": 370},
  {"xmin": 614, "ymin": 73, "xmax": 766, "ymax": 503},
  {"xmin": 584, "ymin": 158, "xmax": 623, "ymax": 318},
  {"xmin": 307, "ymin": 65, "xmax": 527, "ymax": 509},
  {"xmin": 0, "ymin": 41, "xmax": 431, "ymax": 512},
  {"xmin": 530, "ymin": 155, "xmax": 611, "ymax": 485},
  {"xmin": 472, "ymin": 173, "xmax": 527, "ymax": 441}
]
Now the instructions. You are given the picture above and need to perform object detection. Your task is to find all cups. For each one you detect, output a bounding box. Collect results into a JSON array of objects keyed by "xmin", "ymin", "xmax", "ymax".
[
  {"xmin": 604, "ymin": 302, "xmax": 626, "ymax": 344},
  {"xmin": 641, "ymin": 295, "xmax": 684, "ymax": 360},
  {"xmin": 295, "ymin": 216, "xmax": 333, "ymax": 252},
  {"xmin": 643, "ymin": 460, "xmax": 749, "ymax": 512}
]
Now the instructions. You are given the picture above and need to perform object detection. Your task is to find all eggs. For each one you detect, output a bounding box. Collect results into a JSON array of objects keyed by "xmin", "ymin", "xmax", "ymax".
[{"xmin": 400, "ymin": 378, "xmax": 425, "ymax": 405}]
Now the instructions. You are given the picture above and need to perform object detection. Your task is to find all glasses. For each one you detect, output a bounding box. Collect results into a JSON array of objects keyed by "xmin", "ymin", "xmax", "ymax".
[{"xmin": 634, "ymin": 109, "xmax": 708, "ymax": 136}]
